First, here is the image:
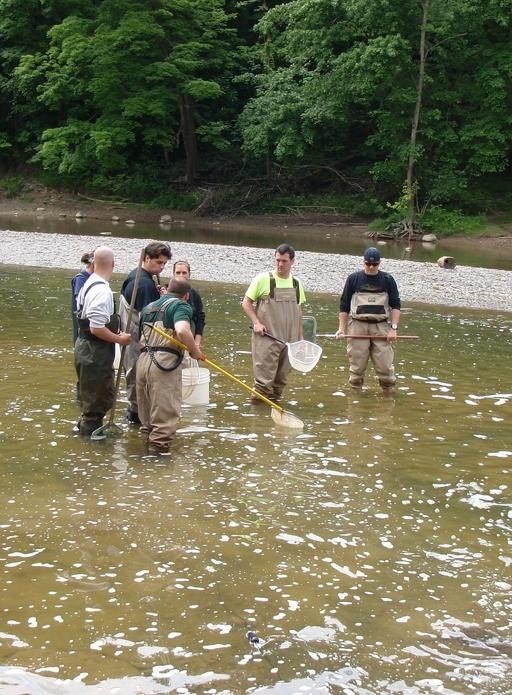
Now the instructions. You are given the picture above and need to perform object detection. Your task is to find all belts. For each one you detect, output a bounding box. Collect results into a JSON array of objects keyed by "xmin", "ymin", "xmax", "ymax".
[
  {"xmin": 352, "ymin": 317, "xmax": 386, "ymax": 324},
  {"xmin": 140, "ymin": 347, "xmax": 179, "ymax": 356}
]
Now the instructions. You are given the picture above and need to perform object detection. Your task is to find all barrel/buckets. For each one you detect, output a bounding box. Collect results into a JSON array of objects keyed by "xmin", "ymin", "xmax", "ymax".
[{"xmin": 181, "ymin": 367, "xmax": 210, "ymax": 404}]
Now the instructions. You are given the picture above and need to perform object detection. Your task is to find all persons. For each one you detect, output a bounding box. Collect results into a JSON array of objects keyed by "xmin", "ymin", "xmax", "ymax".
[
  {"xmin": 116, "ymin": 241, "xmax": 173, "ymax": 423},
  {"xmin": 163, "ymin": 259, "xmax": 206, "ymax": 369},
  {"xmin": 133, "ymin": 278, "xmax": 202, "ymax": 454},
  {"xmin": 73, "ymin": 243, "xmax": 132, "ymax": 442},
  {"xmin": 239, "ymin": 243, "xmax": 308, "ymax": 411},
  {"xmin": 334, "ymin": 247, "xmax": 402, "ymax": 392},
  {"xmin": 69, "ymin": 246, "xmax": 95, "ymax": 405}
]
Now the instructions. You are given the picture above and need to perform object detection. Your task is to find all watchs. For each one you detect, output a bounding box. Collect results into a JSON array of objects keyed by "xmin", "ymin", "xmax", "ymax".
[{"xmin": 390, "ymin": 323, "xmax": 398, "ymax": 330}]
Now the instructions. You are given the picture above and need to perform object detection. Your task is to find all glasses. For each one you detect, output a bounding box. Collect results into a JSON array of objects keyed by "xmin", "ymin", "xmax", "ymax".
[{"xmin": 365, "ymin": 262, "xmax": 379, "ymax": 266}]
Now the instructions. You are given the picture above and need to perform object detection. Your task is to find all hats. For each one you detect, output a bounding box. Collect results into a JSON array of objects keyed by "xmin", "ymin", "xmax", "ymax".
[{"xmin": 364, "ymin": 247, "xmax": 380, "ymax": 262}]
